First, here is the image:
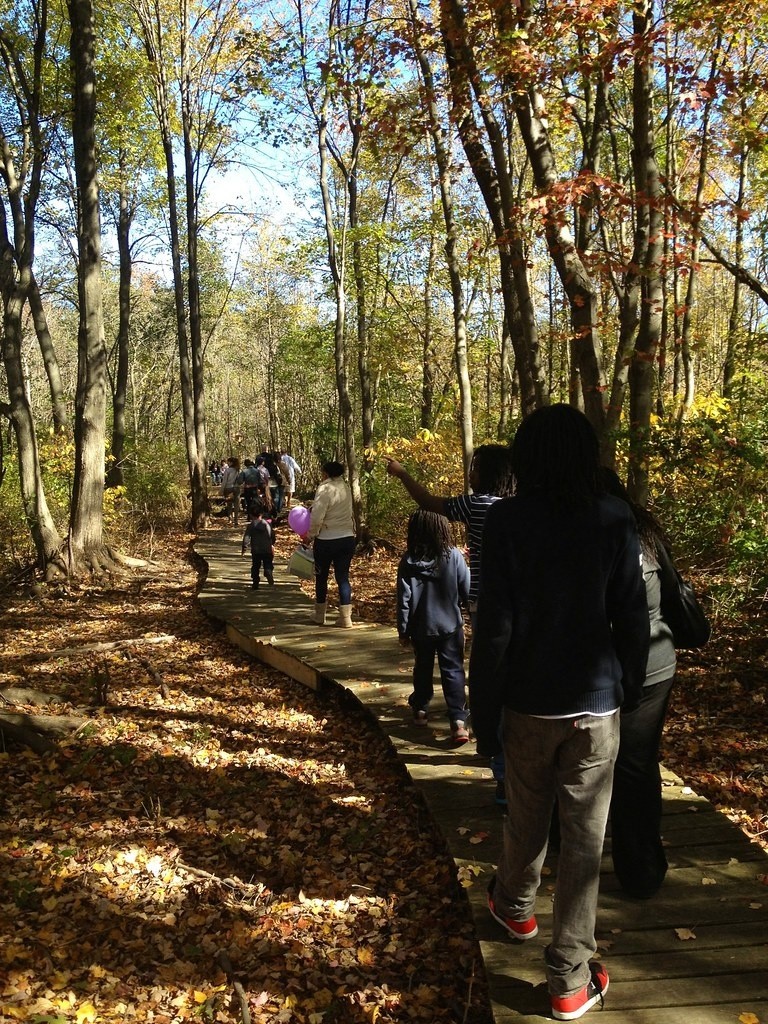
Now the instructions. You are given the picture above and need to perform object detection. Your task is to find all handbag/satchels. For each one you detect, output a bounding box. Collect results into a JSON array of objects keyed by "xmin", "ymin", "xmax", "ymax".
[
  {"xmin": 652, "ymin": 534, "xmax": 711, "ymax": 649},
  {"xmin": 224, "ymin": 489, "xmax": 235, "ymax": 500},
  {"xmin": 287, "ymin": 542, "xmax": 314, "ymax": 580}
]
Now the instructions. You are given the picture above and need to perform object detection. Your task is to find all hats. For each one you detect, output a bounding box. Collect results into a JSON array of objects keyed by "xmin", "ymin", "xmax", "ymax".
[{"xmin": 256, "ymin": 457, "xmax": 266, "ymax": 462}]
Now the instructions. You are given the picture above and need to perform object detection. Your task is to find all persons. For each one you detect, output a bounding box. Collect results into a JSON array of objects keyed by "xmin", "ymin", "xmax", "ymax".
[
  {"xmin": 209, "ymin": 460, "xmax": 229, "ymax": 486},
  {"xmin": 222, "ymin": 446, "xmax": 302, "ymax": 527},
  {"xmin": 395, "ymin": 506, "xmax": 470, "ymax": 744},
  {"xmin": 601, "ymin": 467, "xmax": 677, "ymax": 896},
  {"xmin": 239, "ymin": 505, "xmax": 275, "ymax": 589},
  {"xmin": 383, "ymin": 444, "xmax": 517, "ymax": 762},
  {"xmin": 302, "ymin": 461, "xmax": 356, "ymax": 627},
  {"xmin": 470, "ymin": 402, "xmax": 651, "ymax": 1019}
]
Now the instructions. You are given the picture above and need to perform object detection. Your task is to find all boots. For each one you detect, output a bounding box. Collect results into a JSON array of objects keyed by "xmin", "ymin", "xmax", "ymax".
[
  {"xmin": 310, "ymin": 603, "xmax": 327, "ymax": 625},
  {"xmin": 335, "ymin": 604, "xmax": 352, "ymax": 628}
]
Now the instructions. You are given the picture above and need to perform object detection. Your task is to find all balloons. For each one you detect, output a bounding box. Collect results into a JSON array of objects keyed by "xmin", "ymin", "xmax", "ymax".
[{"xmin": 288, "ymin": 506, "xmax": 310, "ymax": 536}]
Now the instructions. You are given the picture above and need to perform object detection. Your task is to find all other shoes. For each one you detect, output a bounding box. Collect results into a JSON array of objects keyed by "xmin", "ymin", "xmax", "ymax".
[
  {"xmin": 266, "ymin": 573, "xmax": 273, "ymax": 585},
  {"xmin": 252, "ymin": 584, "xmax": 259, "ymax": 590},
  {"xmin": 495, "ymin": 782, "xmax": 508, "ymax": 804}
]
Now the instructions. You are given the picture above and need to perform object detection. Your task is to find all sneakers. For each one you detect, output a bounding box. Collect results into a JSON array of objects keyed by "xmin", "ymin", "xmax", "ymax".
[
  {"xmin": 413, "ymin": 710, "xmax": 427, "ymax": 725},
  {"xmin": 450, "ymin": 720, "xmax": 469, "ymax": 743},
  {"xmin": 487, "ymin": 893, "xmax": 538, "ymax": 940},
  {"xmin": 550, "ymin": 962, "xmax": 609, "ymax": 1020}
]
